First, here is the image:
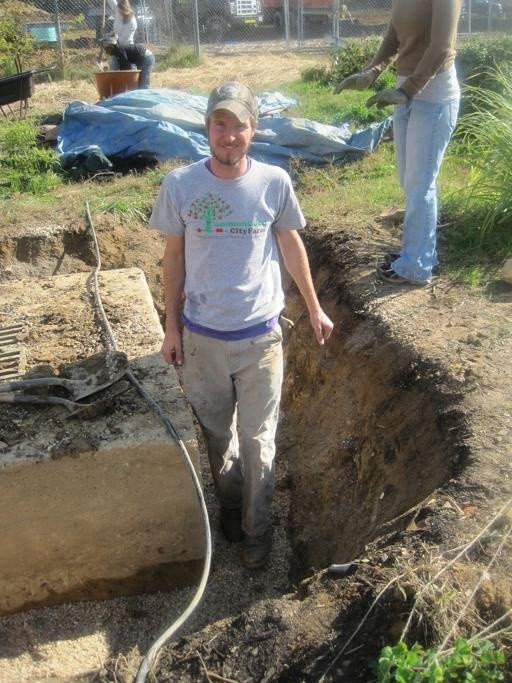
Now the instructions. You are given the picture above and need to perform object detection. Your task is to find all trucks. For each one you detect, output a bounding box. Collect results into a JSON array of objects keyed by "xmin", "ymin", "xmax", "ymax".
[{"xmin": 186, "ymin": 0, "xmax": 336, "ymax": 40}]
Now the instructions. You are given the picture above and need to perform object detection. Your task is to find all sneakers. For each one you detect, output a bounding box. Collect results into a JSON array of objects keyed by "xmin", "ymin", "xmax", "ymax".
[
  {"xmin": 384, "ymin": 253, "xmax": 441, "ymax": 275},
  {"xmin": 377, "ymin": 263, "xmax": 427, "ymax": 284}
]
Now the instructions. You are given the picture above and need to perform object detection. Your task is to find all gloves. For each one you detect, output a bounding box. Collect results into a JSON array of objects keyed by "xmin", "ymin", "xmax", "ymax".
[
  {"xmin": 366, "ymin": 87, "xmax": 408, "ymax": 109},
  {"xmin": 333, "ymin": 69, "xmax": 375, "ymax": 95}
]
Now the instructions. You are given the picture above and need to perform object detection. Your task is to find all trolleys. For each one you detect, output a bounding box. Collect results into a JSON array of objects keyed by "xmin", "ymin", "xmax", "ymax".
[
  {"xmin": 0, "ymin": 61, "xmax": 58, "ymax": 124},
  {"xmin": 91, "ymin": 60, "xmax": 143, "ymax": 99}
]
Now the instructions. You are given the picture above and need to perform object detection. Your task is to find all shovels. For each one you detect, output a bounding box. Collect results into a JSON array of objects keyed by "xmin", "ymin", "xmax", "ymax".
[{"xmin": 0, "ymin": 351, "xmax": 131, "ymax": 420}]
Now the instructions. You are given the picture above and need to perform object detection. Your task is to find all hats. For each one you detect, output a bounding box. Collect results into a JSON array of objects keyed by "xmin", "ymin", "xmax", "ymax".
[{"xmin": 205, "ymin": 79, "xmax": 258, "ymax": 124}]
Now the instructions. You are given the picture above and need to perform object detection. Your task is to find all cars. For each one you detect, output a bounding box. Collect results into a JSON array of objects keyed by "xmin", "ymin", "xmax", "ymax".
[{"xmin": 467, "ymin": 0, "xmax": 504, "ymax": 18}]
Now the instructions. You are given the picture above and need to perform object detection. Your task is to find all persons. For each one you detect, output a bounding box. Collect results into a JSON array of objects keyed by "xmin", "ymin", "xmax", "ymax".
[
  {"xmin": 147, "ymin": 80, "xmax": 337, "ymax": 570},
  {"xmin": 101, "ymin": 38, "xmax": 156, "ymax": 88},
  {"xmin": 103, "ymin": 0, "xmax": 138, "ymax": 70},
  {"xmin": 335, "ymin": 1, "xmax": 463, "ymax": 285}
]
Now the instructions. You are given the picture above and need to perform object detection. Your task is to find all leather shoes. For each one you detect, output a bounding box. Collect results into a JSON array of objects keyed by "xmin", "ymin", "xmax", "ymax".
[
  {"xmin": 220, "ymin": 505, "xmax": 243, "ymax": 543},
  {"xmin": 242, "ymin": 524, "xmax": 273, "ymax": 568}
]
